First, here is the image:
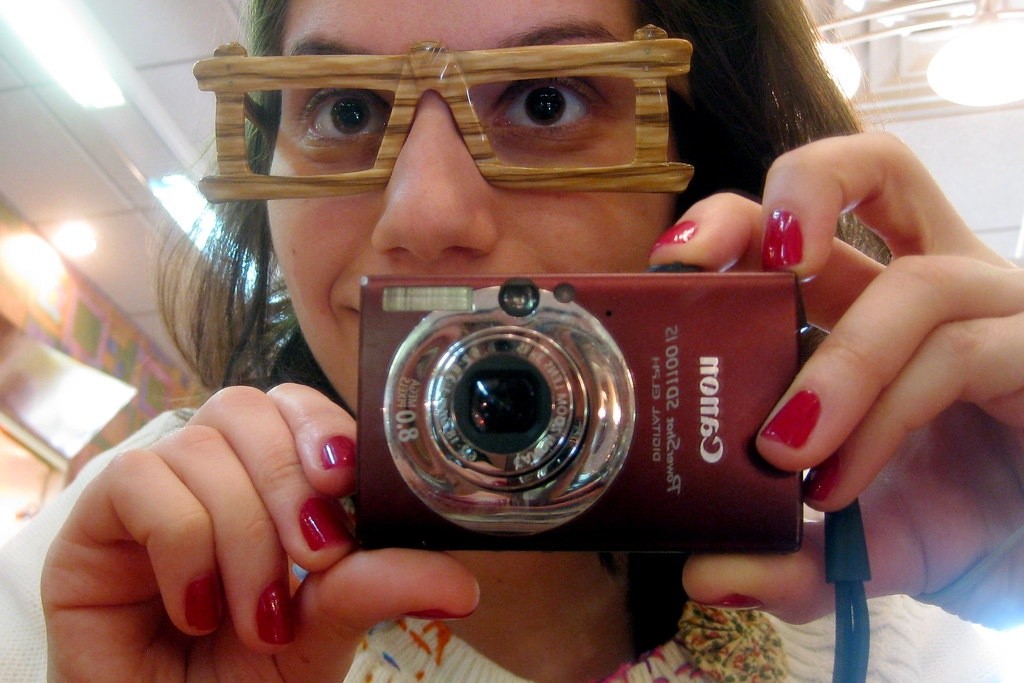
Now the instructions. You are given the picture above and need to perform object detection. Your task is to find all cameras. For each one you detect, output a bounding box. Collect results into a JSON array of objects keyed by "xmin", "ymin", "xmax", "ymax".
[{"xmin": 354, "ymin": 269, "xmax": 805, "ymax": 555}]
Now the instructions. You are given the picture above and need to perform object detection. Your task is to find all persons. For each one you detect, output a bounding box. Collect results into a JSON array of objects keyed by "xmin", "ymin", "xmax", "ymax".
[{"xmin": 1, "ymin": 0, "xmax": 1023, "ymax": 683}]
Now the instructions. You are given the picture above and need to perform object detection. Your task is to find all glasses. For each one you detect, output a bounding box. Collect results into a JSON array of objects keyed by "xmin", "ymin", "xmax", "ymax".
[{"xmin": 191, "ymin": 24, "xmax": 706, "ymax": 205}]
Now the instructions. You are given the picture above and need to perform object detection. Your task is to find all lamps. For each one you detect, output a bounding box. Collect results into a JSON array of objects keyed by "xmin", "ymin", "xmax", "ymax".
[{"xmin": 928, "ymin": 1, "xmax": 1024, "ymax": 109}]
